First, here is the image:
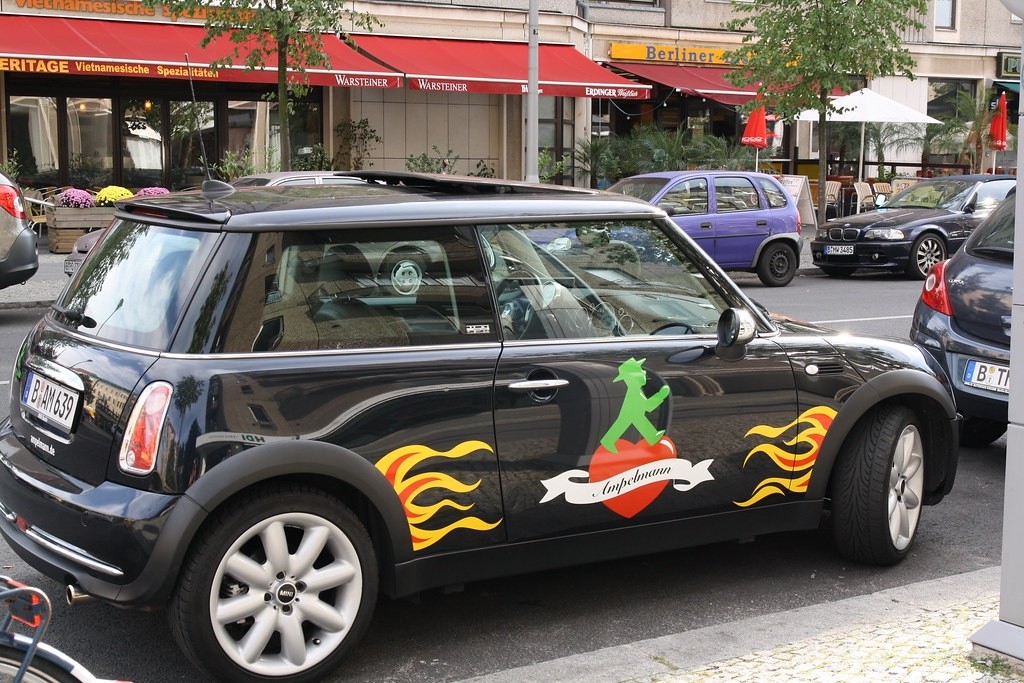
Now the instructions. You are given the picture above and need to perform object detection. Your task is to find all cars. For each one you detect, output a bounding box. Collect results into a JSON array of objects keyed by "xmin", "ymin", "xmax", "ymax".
[
  {"xmin": 811, "ymin": 175, "xmax": 1017, "ymax": 282},
  {"xmin": 64, "ymin": 171, "xmax": 446, "ymax": 295},
  {"xmin": 0, "ymin": 168, "xmax": 39, "ymax": 290},
  {"xmin": 526, "ymin": 169, "xmax": 805, "ymax": 290},
  {"xmin": 908, "ymin": 183, "xmax": 1015, "ymax": 449}
]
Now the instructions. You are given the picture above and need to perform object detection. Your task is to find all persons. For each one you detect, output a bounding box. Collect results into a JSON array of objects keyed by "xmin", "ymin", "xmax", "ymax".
[{"xmin": 597, "ymin": 175, "xmax": 612, "ymax": 190}]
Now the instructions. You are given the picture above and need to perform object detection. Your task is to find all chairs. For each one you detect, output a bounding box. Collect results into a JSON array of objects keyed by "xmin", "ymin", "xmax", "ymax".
[
  {"xmin": 807, "ymin": 175, "xmax": 890, "ymax": 214},
  {"xmin": 366, "ymin": 244, "xmax": 462, "ymax": 343},
  {"xmin": 24, "ymin": 186, "xmax": 101, "ymax": 238},
  {"xmin": 518, "ymin": 281, "xmax": 592, "ymax": 340},
  {"xmin": 311, "ymin": 245, "xmax": 397, "ymax": 347}
]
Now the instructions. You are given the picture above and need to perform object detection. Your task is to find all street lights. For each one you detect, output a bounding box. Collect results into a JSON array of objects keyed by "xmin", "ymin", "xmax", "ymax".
[
  {"xmin": 67, "ymin": 358, "xmax": 92, "ymax": 369},
  {"xmin": 96, "ymin": 298, "xmax": 124, "ymax": 335}
]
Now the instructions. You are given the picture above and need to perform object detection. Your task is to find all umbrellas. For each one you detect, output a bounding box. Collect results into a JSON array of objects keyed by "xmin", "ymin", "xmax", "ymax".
[
  {"xmin": 986, "ymin": 91, "xmax": 1008, "ymax": 175},
  {"xmin": 779, "ymin": 88, "xmax": 944, "ymax": 215},
  {"xmin": 741, "ymin": 85, "xmax": 768, "ymax": 172}
]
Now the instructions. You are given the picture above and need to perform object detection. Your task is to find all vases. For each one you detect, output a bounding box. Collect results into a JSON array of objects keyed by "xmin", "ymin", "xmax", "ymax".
[{"xmin": 45, "ymin": 206, "xmax": 115, "ymax": 228}]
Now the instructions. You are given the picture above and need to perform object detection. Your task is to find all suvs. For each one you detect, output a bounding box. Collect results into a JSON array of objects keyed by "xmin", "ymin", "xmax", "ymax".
[{"xmin": 0, "ymin": 165, "xmax": 964, "ymax": 683}]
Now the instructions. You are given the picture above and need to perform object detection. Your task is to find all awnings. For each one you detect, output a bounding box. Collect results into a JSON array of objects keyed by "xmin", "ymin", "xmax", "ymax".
[
  {"xmin": 603, "ymin": 62, "xmax": 856, "ymax": 106},
  {"xmin": 0, "ymin": 12, "xmax": 405, "ymax": 89},
  {"xmin": 992, "ymin": 80, "xmax": 1020, "ymax": 93},
  {"xmin": 341, "ymin": 31, "xmax": 653, "ymax": 99}
]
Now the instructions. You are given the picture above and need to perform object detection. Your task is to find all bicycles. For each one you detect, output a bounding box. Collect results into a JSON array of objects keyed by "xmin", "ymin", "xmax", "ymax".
[{"xmin": 0, "ymin": 576, "xmax": 137, "ymax": 682}]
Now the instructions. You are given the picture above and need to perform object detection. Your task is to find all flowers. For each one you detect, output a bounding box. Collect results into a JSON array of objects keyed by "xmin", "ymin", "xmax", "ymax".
[{"xmin": 58, "ymin": 185, "xmax": 170, "ymax": 209}]
